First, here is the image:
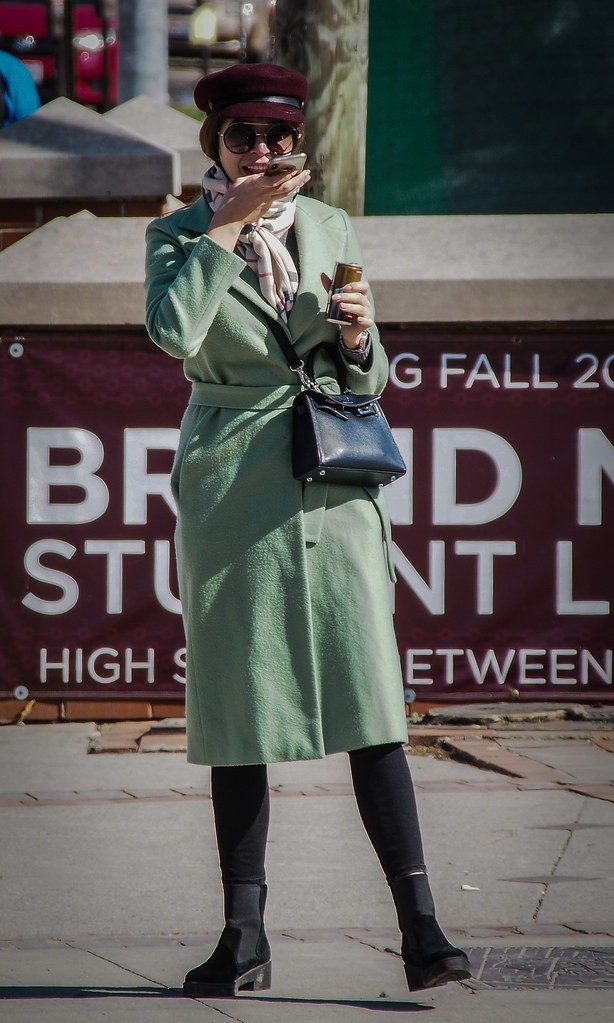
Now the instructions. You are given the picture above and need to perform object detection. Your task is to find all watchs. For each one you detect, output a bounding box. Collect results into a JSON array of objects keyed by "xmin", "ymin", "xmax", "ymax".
[{"xmin": 340, "ymin": 329, "xmax": 368, "ymax": 352}]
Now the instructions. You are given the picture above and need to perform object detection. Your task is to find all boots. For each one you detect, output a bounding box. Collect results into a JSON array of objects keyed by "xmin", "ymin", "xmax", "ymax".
[
  {"xmin": 183, "ymin": 882, "xmax": 272, "ymax": 996},
  {"xmin": 389, "ymin": 874, "xmax": 471, "ymax": 991}
]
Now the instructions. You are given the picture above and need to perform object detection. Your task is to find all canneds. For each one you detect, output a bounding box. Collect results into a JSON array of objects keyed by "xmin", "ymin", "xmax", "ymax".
[{"xmin": 324, "ymin": 261, "xmax": 363, "ymax": 324}]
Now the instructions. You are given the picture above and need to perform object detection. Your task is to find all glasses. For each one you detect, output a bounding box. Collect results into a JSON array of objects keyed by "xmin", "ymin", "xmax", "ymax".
[{"xmin": 217, "ymin": 121, "xmax": 302, "ymax": 156}]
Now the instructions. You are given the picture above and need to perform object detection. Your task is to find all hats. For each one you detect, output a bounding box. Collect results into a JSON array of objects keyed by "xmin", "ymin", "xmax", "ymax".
[{"xmin": 193, "ymin": 62, "xmax": 308, "ymax": 124}]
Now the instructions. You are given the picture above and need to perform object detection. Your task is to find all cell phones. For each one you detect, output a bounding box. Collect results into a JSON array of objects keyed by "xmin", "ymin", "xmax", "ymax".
[{"xmin": 263, "ymin": 152, "xmax": 307, "ymax": 177}]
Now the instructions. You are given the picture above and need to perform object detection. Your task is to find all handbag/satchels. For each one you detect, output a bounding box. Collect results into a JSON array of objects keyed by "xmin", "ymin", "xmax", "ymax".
[{"xmin": 292, "ymin": 390, "xmax": 407, "ymax": 487}]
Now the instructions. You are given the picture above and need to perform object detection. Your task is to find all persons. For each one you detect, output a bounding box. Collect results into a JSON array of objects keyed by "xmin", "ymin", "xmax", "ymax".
[{"xmin": 143, "ymin": 64, "xmax": 471, "ymax": 996}]
